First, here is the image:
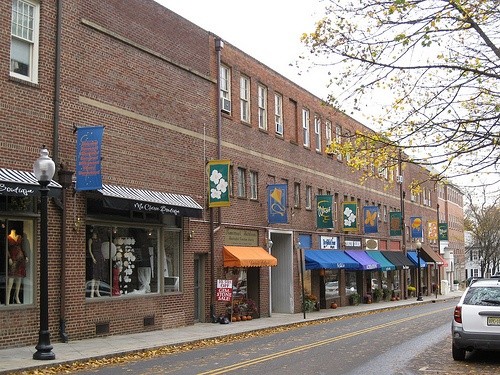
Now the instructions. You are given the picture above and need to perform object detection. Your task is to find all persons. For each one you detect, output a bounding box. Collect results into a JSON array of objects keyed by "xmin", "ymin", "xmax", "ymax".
[
  {"xmin": 89, "ymin": 233, "xmax": 105, "ymax": 297},
  {"xmin": 7, "ymin": 229, "xmax": 29, "ymax": 305},
  {"xmin": 136, "ymin": 243, "xmax": 152, "ymax": 293}
]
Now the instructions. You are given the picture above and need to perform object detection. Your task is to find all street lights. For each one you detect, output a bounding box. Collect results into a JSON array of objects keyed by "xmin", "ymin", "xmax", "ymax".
[
  {"xmin": 32, "ymin": 143, "xmax": 56, "ymax": 361},
  {"xmin": 415, "ymin": 239, "xmax": 424, "ymax": 301}
]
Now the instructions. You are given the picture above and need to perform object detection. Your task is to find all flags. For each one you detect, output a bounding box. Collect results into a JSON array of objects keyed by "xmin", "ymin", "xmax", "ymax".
[
  {"xmin": 439, "ymin": 223, "xmax": 448, "ymax": 239},
  {"xmin": 428, "ymin": 220, "xmax": 438, "ymax": 239},
  {"xmin": 410, "ymin": 217, "xmax": 422, "ymax": 237},
  {"xmin": 208, "ymin": 159, "xmax": 231, "ymax": 207},
  {"xmin": 342, "ymin": 201, "xmax": 359, "ymax": 231},
  {"xmin": 267, "ymin": 183, "xmax": 290, "ymax": 224},
  {"xmin": 316, "ymin": 195, "xmax": 334, "ymax": 229},
  {"xmin": 75, "ymin": 126, "xmax": 105, "ymax": 190},
  {"xmin": 389, "ymin": 212, "xmax": 402, "ymax": 236},
  {"xmin": 363, "ymin": 206, "xmax": 379, "ymax": 233}
]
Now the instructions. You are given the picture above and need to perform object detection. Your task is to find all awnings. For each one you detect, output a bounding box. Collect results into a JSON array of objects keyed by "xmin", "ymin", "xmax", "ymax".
[
  {"xmin": 0, "ymin": 167, "xmax": 63, "ymax": 197},
  {"xmin": 305, "ymin": 243, "xmax": 449, "ymax": 270},
  {"xmin": 71, "ymin": 180, "xmax": 204, "ymax": 219},
  {"xmin": 222, "ymin": 246, "xmax": 277, "ymax": 268}
]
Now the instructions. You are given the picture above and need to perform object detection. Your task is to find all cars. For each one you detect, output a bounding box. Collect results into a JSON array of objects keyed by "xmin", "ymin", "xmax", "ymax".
[{"xmin": 451, "ymin": 272, "xmax": 500, "ymax": 361}]
